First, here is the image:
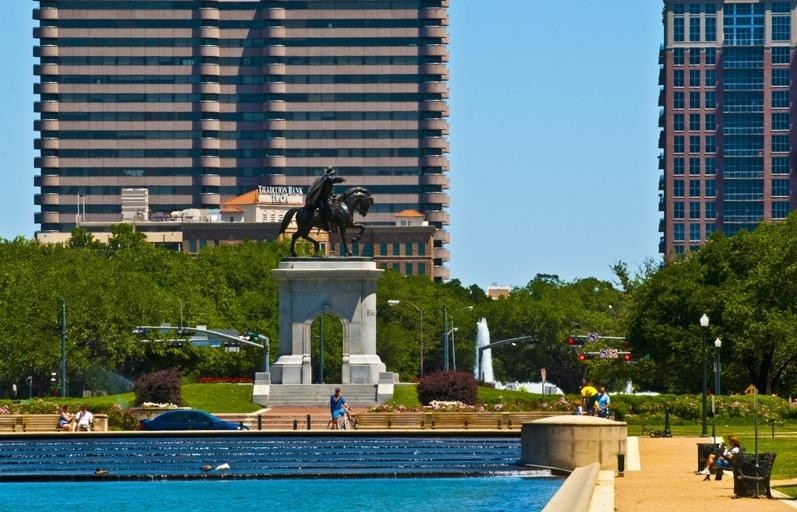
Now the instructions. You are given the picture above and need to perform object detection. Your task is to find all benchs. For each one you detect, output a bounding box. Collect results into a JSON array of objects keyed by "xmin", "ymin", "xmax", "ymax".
[
  {"xmin": 733, "ymin": 452, "xmax": 776, "ymax": 500},
  {"xmin": 703, "ymin": 448, "xmax": 747, "ymax": 481},
  {"xmin": 351, "ymin": 412, "xmax": 427, "ymax": 431},
  {"xmin": 430, "ymin": 414, "xmax": 504, "ymax": 430},
  {"xmin": 508, "ymin": 414, "xmax": 555, "ymax": 429},
  {"xmin": 22, "ymin": 415, "xmax": 97, "ymax": 432},
  {"xmin": 0, "ymin": 414, "xmax": 16, "ymax": 432}
]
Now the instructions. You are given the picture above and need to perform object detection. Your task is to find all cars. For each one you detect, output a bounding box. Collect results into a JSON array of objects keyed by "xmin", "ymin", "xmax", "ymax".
[{"xmin": 137, "ymin": 407, "xmax": 251, "ymax": 430}]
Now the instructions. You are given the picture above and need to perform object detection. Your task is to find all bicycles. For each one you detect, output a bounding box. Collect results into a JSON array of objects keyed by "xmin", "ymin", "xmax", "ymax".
[{"xmin": 326, "ymin": 405, "xmax": 354, "ymax": 429}]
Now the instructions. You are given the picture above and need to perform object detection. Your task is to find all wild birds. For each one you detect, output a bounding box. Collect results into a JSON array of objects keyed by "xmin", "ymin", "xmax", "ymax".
[
  {"xmin": 201, "ymin": 462, "xmax": 230, "ymax": 474},
  {"xmin": 95, "ymin": 469, "xmax": 109, "ymax": 476}
]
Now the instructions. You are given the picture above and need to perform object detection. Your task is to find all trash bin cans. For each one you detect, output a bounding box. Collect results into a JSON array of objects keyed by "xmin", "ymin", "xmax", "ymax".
[{"xmin": 697, "ymin": 443, "xmax": 720, "ymax": 472}]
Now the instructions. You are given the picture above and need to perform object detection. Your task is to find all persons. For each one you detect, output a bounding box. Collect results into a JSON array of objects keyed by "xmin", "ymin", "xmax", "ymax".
[
  {"xmin": 75, "ymin": 404, "xmax": 93, "ymax": 431},
  {"xmin": 305, "ymin": 165, "xmax": 347, "ymax": 234},
  {"xmin": 593, "ymin": 386, "xmax": 611, "ymax": 418},
  {"xmin": 574, "ymin": 400, "xmax": 582, "ymax": 415},
  {"xmin": 576, "ymin": 385, "xmax": 599, "ymax": 416},
  {"xmin": 330, "ymin": 388, "xmax": 352, "ymax": 430},
  {"xmin": 696, "ymin": 435, "xmax": 740, "ymax": 475},
  {"xmin": 58, "ymin": 405, "xmax": 74, "ymax": 431}
]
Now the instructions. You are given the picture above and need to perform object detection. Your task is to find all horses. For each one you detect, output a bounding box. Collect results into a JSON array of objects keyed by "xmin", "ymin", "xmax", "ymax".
[{"xmin": 276, "ymin": 185, "xmax": 375, "ymax": 257}]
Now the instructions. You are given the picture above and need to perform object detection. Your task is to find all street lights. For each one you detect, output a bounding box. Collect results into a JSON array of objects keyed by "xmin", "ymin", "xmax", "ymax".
[
  {"xmin": 442, "ymin": 325, "xmax": 460, "ymax": 374},
  {"xmin": 713, "ymin": 336, "xmax": 722, "ymax": 393},
  {"xmin": 450, "ymin": 304, "xmax": 475, "ymax": 372},
  {"xmin": 698, "ymin": 312, "xmax": 709, "ymax": 437},
  {"xmin": 386, "ymin": 297, "xmax": 423, "ymax": 379},
  {"xmin": 608, "ymin": 304, "xmax": 613, "ymax": 337}
]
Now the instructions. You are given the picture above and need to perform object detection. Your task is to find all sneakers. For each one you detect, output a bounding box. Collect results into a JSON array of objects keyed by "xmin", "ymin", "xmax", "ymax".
[{"xmin": 699, "ymin": 469, "xmax": 710, "ymax": 475}]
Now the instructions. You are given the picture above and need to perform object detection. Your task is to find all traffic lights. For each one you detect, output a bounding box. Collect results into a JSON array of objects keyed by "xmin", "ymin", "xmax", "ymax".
[
  {"xmin": 566, "ymin": 337, "xmax": 584, "ymax": 346},
  {"xmin": 578, "ymin": 354, "xmax": 594, "ymax": 361},
  {"xmin": 623, "ymin": 354, "xmax": 631, "ymax": 361}
]
